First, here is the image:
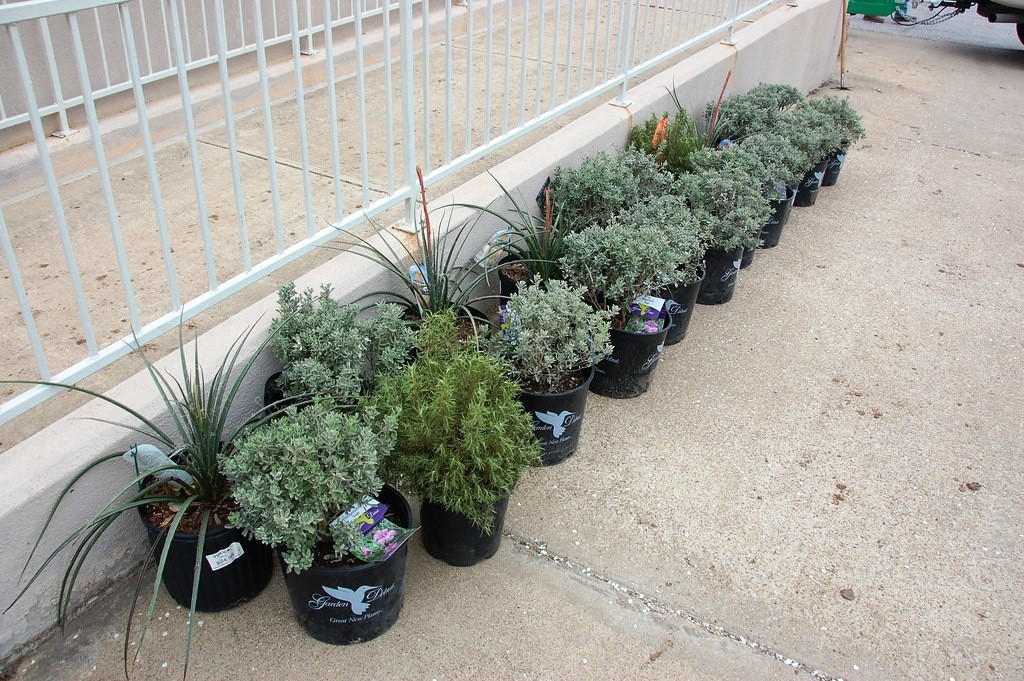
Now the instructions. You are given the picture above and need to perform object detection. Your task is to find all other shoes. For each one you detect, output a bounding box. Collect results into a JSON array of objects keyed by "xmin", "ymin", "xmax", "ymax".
[
  {"xmin": 863, "ymin": 14, "xmax": 885, "ymax": 24},
  {"xmin": 893, "ymin": 11, "xmax": 917, "ymax": 23}
]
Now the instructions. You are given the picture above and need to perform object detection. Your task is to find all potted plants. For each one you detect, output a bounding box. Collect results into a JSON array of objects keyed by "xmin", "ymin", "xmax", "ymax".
[{"xmin": 0, "ymin": 66, "xmax": 867, "ymax": 681}]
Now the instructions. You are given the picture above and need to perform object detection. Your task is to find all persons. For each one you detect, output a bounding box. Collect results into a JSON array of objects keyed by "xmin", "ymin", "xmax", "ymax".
[{"xmin": 863, "ymin": 0, "xmax": 917, "ymax": 23}]
